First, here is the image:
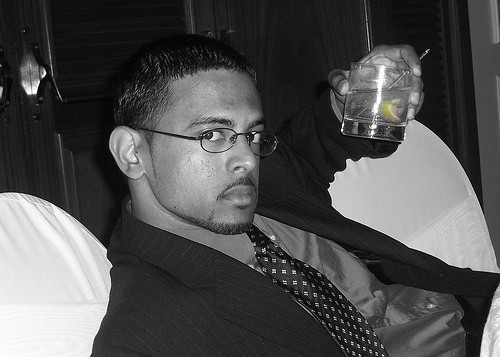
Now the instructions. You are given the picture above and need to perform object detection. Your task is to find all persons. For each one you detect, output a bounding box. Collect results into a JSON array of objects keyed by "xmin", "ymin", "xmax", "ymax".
[{"xmin": 92, "ymin": 32, "xmax": 500, "ymax": 356}]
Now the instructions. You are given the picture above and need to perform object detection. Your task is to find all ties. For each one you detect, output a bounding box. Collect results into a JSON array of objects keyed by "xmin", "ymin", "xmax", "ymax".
[{"xmin": 245, "ymin": 223, "xmax": 390, "ymax": 357}]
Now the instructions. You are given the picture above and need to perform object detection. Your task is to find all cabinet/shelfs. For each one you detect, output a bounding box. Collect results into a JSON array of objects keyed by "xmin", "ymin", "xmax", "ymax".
[{"xmin": 0, "ymin": 0, "xmax": 484, "ymax": 247}]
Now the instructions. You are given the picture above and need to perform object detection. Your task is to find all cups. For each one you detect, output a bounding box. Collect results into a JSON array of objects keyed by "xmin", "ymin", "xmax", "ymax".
[{"xmin": 328, "ymin": 62, "xmax": 414, "ymax": 142}]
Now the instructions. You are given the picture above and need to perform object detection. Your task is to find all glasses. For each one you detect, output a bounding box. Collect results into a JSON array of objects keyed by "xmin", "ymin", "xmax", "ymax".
[{"xmin": 133, "ymin": 127, "xmax": 280, "ymax": 157}]
